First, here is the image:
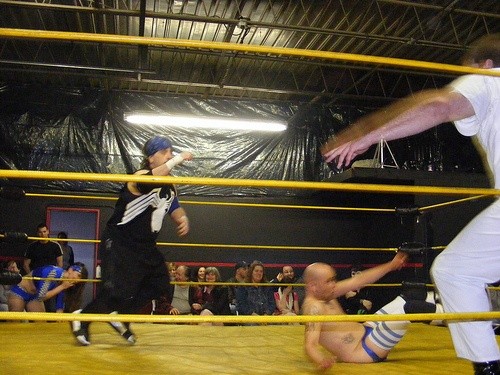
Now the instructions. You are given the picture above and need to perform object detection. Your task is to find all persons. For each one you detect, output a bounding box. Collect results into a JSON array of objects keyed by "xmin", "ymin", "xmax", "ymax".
[
  {"xmin": 344, "ymin": 264, "xmax": 372, "ymax": 314},
  {"xmin": 23, "ymin": 223, "xmax": 64, "ymax": 274},
  {"xmin": 0, "ymin": 260, "xmax": 26, "ymax": 311},
  {"xmin": 57, "ymin": 231, "xmax": 74, "ymax": 267},
  {"xmin": 7, "ymin": 262, "xmax": 88, "ymax": 323},
  {"xmin": 149, "ymin": 258, "xmax": 300, "ymax": 327},
  {"xmin": 324, "ymin": 37, "xmax": 500, "ymax": 375},
  {"xmin": 69, "ymin": 136, "xmax": 193, "ymax": 345},
  {"xmin": 300, "ymin": 252, "xmax": 443, "ymax": 375}
]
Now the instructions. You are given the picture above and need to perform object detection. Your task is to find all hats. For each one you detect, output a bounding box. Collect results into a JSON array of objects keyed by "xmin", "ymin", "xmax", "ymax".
[
  {"xmin": 141, "ymin": 135, "xmax": 173, "ymax": 157},
  {"xmin": 234, "ymin": 261, "xmax": 250, "ymax": 269}
]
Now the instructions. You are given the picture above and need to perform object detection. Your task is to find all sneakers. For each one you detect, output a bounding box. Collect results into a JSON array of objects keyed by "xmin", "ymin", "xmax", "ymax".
[
  {"xmin": 105, "ymin": 310, "xmax": 137, "ymax": 345},
  {"xmin": 68, "ymin": 308, "xmax": 91, "ymax": 346}
]
